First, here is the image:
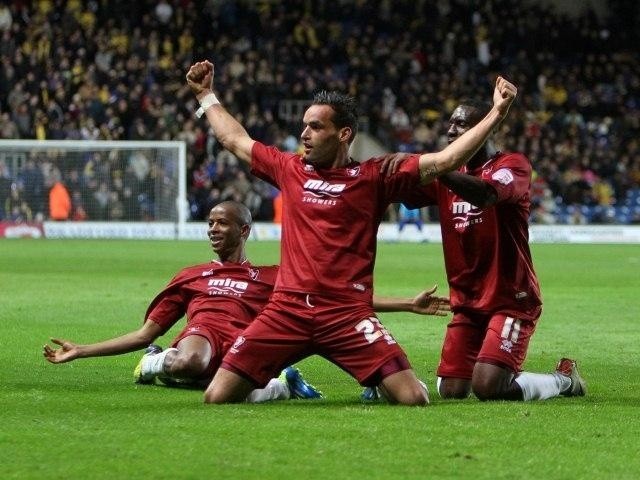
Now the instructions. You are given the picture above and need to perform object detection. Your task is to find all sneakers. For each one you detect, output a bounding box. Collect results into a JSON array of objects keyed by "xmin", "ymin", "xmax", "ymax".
[
  {"xmin": 362, "ymin": 387, "xmax": 381, "ymax": 401},
  {"xmin": 133, "ymin": 345, "xmax": 162, "ymax": 384},
  {"xmin": 556, "ymin": 356, "xmax": 585, "ymax": 396},
  {"xmin": 278, "ymin": 365, "xmax": 324, "ymax": 398}
]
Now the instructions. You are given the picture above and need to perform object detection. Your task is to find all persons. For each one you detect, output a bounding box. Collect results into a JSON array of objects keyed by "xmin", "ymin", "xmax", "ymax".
[
  {"xmin": 1, "ymin": 0, "xmax": 639, "ymax": 243},
  {"xmin": 186, "ymin": 59, "xmax": 519, "ymax": 407},
  {"xmin": 374, "ymin": 98, "xmax": 587, "ymax": 402},
  {"xmin": 42, "ymin": 200, "xmax": 452, "ymax": 389}
]
